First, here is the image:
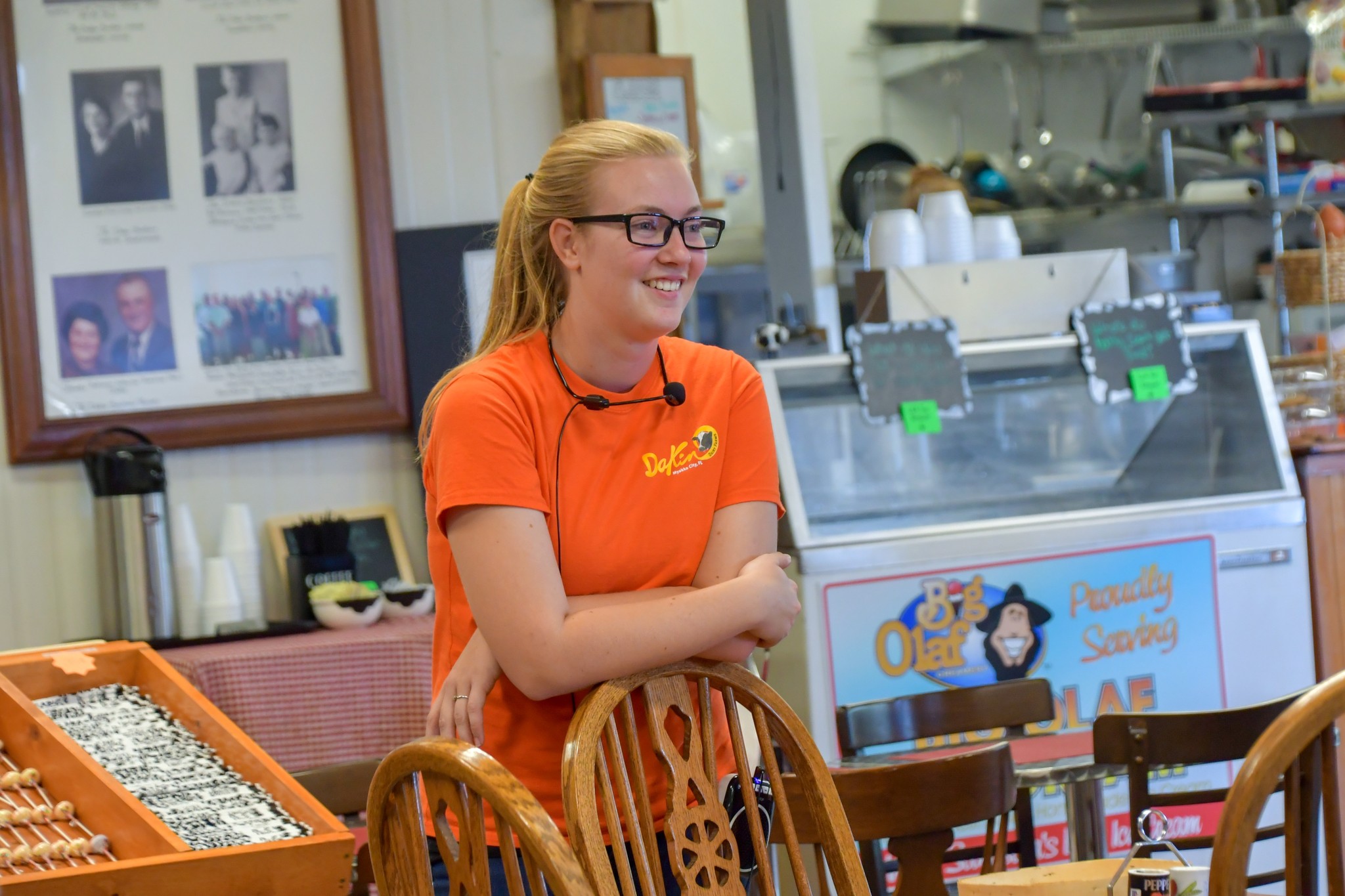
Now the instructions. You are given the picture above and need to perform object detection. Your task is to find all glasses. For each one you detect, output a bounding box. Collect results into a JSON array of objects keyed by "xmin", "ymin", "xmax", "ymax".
[{"xmin": 568, "ymin": 210, "xmax": 726, "ymax": 249}]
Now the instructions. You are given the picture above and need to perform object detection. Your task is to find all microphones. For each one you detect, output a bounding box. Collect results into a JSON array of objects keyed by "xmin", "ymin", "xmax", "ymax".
[{"xmin": 580, "ymin": 379, "xmax": 686, "ymax": 413}]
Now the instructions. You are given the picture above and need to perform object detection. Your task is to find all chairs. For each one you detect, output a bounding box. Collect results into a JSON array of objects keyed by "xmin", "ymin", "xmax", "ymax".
[
  {"xmin": 558, "ymin": 658, "xmax": 874, "ymax": 896},
  {"xmin": 832, "ymin": 676, "xmax": 1056, "ymax": 896},
  {"xmin": 362, "ymin": 734, "xmax": 600, "ymax": 896},
  {"xmin": 1091, "ymin": 669, "xmax": 1345, "ymax": 896}
]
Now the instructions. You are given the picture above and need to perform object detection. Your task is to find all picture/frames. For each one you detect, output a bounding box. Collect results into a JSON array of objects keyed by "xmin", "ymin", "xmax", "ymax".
[
  {"xmin": 581, "ymin": 49, "xmax": 699, "ymax": 196},
  {"xmin": 1, "ymin": 1, "xmax": 407, "ymax": 466}
]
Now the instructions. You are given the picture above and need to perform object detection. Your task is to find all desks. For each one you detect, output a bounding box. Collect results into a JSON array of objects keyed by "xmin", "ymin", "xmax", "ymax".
[
  {"xmin": 842, "ymin": 727, "xmax": 1129, "ymax": 862},
  {"xmin": 159, "ymin": 603, "xmax": 449, "ymax": 804}
]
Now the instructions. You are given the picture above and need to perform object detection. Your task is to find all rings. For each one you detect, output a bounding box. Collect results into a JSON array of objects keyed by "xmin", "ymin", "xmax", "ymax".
[{"xmin": 453, "ymin": 695, "xmax": 469, "ymax": 700}]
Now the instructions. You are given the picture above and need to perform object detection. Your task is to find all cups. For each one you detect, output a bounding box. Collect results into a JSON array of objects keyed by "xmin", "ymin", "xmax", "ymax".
[{"xmin": 167, "ymin": 503, "xmax": 269, "ymax": 641}]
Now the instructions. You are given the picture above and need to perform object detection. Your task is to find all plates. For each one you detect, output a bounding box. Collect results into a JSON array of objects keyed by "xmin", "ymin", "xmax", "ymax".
[{"xmin": 842, "ymin": 142, "xmax": 922, "ymax": 240}]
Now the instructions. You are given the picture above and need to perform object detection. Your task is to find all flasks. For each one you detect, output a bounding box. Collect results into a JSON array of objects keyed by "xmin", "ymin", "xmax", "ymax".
[{"xmin": 84, "ymin": 426, "xmax": 179, "ymax": 646}]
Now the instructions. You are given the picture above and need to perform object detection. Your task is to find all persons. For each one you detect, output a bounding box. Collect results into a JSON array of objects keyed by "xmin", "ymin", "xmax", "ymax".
[
  {"xmin": 192, "ymin": 283, "xmax": 343, "ymax": 366},
  {"xmin": 200, "ymin": 121, "xmax": 252, "ymax": 195},
  {"xmin": 213, "ymin": 62, "xmax": 262, "ymax": 157},
  {"xmin": 242, "ymin": 112, "xmax": 293, "ymax": 194},
  {"xmin": 108, "ymin": 273, "xmax": 177, "ymax": 374},
  {"xmin": 418, "ymin": 118, "xmax": 804, "ymax": 896},
  {"xmin": 57, "ymin": 299, "xmax": 122, "ymax": 378},
  {"xmin": 111, "ymin": 72, "xmax": 171, "ymax": 202},
  {"xmin": 76, "ymin": 92, "xmax": 137, "ymax": 204}
]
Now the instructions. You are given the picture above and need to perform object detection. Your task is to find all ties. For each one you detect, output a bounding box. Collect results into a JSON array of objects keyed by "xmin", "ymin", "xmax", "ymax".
[
  {"xmin": 136, "ymin": 122, "xmax": 143, "ymax": 148},
  {"xmin": 130, "ymin": 333, "xmax": 140, "ymax": 370}
]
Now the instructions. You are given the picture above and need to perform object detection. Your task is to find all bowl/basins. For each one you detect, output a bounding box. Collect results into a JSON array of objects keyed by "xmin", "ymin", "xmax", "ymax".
[
  {"xmin": 377, "ymin": 584, "xmax": 436, "ymax": 618},
  {"xmin": 861, "ymin": 190, "xmax": 1022, "ymax": 268},
  {"xmin": 311, "ymin": 591, "xmax": 384, "ymax": 628}
]
